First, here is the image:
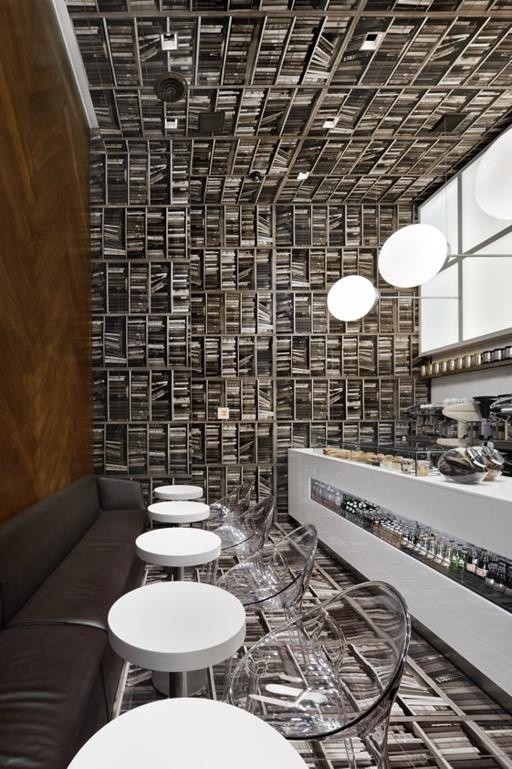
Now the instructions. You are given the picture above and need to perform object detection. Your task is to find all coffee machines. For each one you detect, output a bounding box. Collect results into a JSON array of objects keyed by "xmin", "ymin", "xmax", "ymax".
[{"xmin": 406, "ymin": 393, "xmax": 512, "ymax": 453}]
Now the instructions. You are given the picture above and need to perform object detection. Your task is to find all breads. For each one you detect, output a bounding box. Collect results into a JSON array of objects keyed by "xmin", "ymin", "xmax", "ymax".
[{"xmin": 324, "ymin": 450, "xmax": 415, "ymax": 466}]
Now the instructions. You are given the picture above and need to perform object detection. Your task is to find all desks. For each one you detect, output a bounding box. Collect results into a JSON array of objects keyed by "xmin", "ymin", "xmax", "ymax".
[
  {"xmin": 153, "ymin": 485, "xmax": 204, "ymax": 527},
  {"xmin": 147, "ymin": 501, "xmax": 207, "ymax": 572},
  {"xmin": 134, "ymin": 527, "xmax": 222, "ymax": 697},
  {"xmin": 107, "ymin": 580, "xmax": 247, "ymax": 700},
  {"xmin": 67, "ymin": 695, "xmax": 309, "ymax": 769}
]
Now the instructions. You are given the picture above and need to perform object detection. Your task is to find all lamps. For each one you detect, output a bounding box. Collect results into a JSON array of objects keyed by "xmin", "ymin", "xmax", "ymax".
[
  {"xmin": 376, "ymin": 225, "xmax": 510, "ymax": 288},
  {"xmin": 326, "ymin": 276, "xmax": 461, "ymax": 321}
]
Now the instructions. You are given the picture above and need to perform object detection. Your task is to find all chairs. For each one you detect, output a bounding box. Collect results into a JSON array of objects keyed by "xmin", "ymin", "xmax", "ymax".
[
  {"xmin": 221, "ymin": 581, "xmax": 415, "ymax": 767},
  {"xmin": 212, "ymin": 496, "xmax": 278, "ymax": 595},
  {"xmin": 204, "ymin": 477, "xmax": 255, "ymax": 558},
  {"xmin": 215, "ymin": 522, "xmax": 320, "ymax": 689}
]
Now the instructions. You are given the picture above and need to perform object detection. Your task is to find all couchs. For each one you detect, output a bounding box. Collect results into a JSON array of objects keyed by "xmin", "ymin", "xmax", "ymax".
[{"xmin": 0, "ymin": 474, "xmax": 151, "ymax": 768}]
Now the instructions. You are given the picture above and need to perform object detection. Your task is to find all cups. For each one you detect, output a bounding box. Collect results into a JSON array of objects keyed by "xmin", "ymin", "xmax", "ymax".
[{"xmin": 369, "ymin": 456, "xmax": 429, "ymax": 477}]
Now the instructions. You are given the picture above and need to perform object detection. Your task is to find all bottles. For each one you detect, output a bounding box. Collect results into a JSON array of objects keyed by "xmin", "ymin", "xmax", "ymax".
[
  {"xmin": 420, "ymin": 346, "xmax": 511, "ymax": 376},
  {"xmin": 312, "ymin": 481, "xmax": 508, "ymax": 605}
]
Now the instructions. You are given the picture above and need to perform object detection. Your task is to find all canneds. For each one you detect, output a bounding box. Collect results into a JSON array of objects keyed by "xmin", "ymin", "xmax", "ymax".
[{"xmin": 420, "ymin": 346, "xmax": 512, "ymax": 378}]
